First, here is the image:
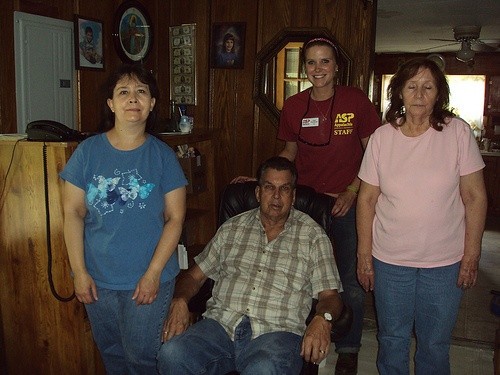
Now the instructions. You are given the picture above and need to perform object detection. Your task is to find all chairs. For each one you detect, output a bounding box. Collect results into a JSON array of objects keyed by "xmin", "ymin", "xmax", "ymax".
[{"xmin": 186, "ymin": 181, "xmax": 354, "ymax": 375}]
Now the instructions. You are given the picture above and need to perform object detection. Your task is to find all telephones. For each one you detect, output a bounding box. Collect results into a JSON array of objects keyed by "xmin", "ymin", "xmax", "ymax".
[{"xmin": 25, "ymin": 119, "xmax": 86, "ymax": 144}]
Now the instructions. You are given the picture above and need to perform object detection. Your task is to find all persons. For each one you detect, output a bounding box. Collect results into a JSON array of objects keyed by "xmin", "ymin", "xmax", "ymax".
[
  {"xmin": 156, "ymin": 158, "xmax": 345, "ymax": 375},
  {"xmin": 353, "ymin": 57, "xmax": 489, "ymax": 375},
  {"xmin": 227, "ymin": 36, "xmax": 379, "ymax": 375},
  {"xmin": 55, "ymin": 62, "xmax": 190, "ymax": 375}
]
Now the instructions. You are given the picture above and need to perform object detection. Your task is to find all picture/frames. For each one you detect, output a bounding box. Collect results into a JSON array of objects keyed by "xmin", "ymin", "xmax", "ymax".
[
  {"xmin": 209, "ymin": 21, "xmax": 246, "ymax": 71},
  {"xmin": 73, "ymin": 14, "xmax": 106, "ymax": 72},
  {"xmin": 111, "ymin": 0, "xmax": 155, "ymax": 66}
]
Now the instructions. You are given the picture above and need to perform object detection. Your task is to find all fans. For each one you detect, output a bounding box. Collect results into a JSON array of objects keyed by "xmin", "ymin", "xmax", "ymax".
[{"xmin": 415, "ymin": 25, "xmax": 500, "ymax": 53}]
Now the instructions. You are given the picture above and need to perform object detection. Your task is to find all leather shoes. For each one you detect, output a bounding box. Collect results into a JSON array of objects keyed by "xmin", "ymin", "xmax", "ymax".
[{"xmin": 335, "ymin": 354, "xmax": 358, "ymax": 375}]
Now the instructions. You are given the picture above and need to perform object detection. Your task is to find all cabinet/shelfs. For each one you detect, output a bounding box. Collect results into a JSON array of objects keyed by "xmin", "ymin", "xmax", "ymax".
[{"xmin": 159, "ymin": 127, "xmax": 216, "ymax": 272}]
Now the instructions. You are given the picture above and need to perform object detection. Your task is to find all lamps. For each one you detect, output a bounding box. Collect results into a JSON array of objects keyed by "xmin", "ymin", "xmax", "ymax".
[{"xmin": 452, "ymin": 25, "xmax": 482, "ymax": 67}]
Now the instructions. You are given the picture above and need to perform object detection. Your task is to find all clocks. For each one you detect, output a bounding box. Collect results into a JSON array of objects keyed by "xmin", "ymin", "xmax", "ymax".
[{"xmin": 426, "ymin": 53, "xmax": 447, "ymax": 73}]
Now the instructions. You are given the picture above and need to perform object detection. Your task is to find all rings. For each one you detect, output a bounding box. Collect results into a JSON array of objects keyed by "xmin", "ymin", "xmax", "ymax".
[
  {"xmin": 462, "ymin": 283, "xmax": 468, "ymax": 287},
  {"xmin": 164, "ymin": 331, "xmax": 169, "ymax": 334}
]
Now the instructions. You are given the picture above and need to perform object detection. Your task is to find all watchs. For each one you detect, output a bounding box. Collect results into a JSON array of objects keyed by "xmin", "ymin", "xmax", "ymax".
[{"xmin": 314, "ymin": 312, "xmax": 334, "ymax": 330}]
